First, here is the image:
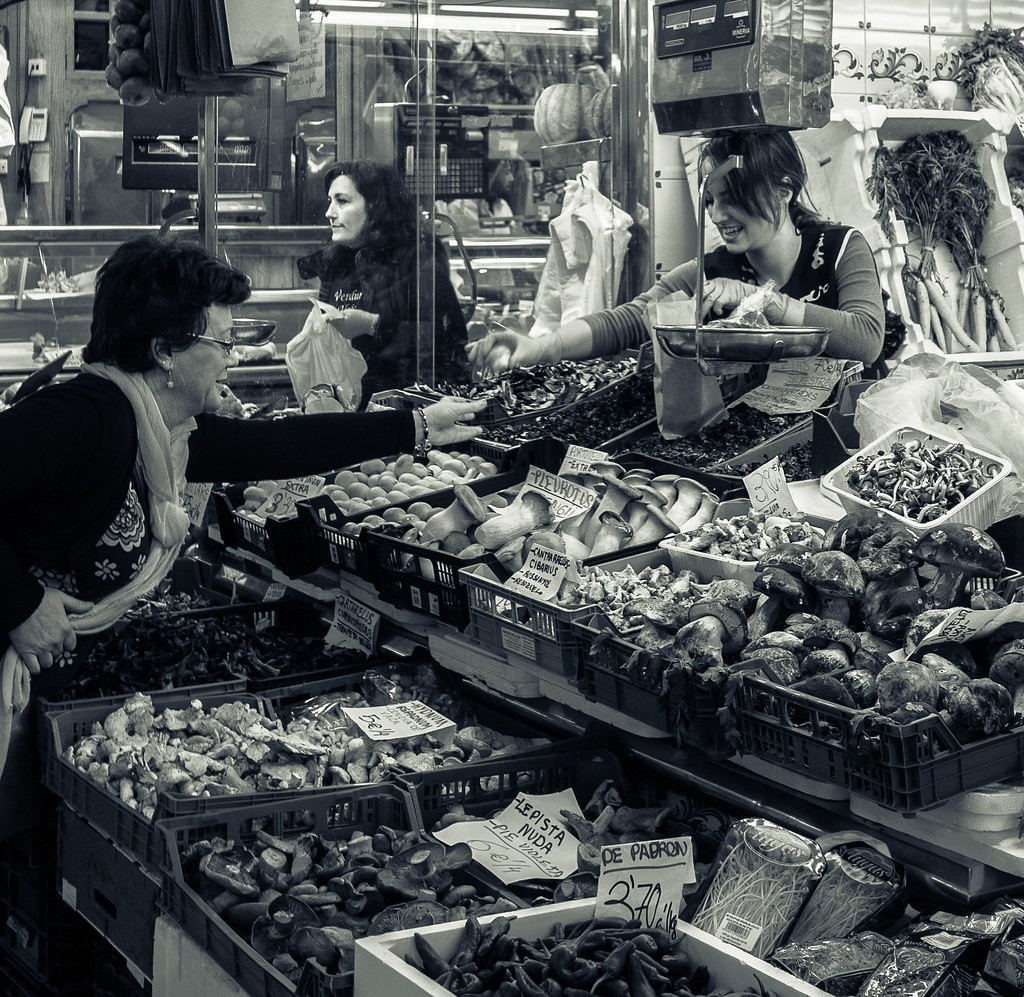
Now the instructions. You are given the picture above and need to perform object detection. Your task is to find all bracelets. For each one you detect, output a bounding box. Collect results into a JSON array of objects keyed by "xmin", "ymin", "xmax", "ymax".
[{"xmin": 413, "ymin": 405, "xmax": 431, "ymax": 461}]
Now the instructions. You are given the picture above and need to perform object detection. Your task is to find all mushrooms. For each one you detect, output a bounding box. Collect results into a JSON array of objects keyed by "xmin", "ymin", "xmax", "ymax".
[{"xmin": 59, "ymin": 435, "xmax": 1024, "ymax": 997}]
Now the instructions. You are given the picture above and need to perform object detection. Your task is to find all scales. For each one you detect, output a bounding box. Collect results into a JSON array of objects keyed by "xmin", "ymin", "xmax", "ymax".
[
  {"xmin": 158, "ymin": 189, "xmax": 268, "ymax": 223},
  {"xmin": 650, "ymin": 0, "xmax": 836, "ymax": 372}
]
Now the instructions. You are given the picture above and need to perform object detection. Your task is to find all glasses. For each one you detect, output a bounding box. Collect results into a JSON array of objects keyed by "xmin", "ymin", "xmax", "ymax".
[{"xmin": 187, "ymin": 332, "xmax": 235, "ymax": 356}]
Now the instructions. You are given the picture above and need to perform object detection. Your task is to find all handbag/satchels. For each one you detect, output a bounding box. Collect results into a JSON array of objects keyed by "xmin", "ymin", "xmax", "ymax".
[{"xmin": 285, "ymin": 297, "xmax": 368, "ymax": 412}]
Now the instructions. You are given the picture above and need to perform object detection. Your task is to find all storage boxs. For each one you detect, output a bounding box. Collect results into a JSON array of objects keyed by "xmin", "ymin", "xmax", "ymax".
[{"xmin": 5, "ymin": 107, "xmax": 1024, "ymax": 997}]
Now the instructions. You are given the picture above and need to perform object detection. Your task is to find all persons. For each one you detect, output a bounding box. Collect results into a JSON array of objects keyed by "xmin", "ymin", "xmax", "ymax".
[
  {"xmin": 0, "ymin": 234, "xmax": 491, "ymax": 996},
  {"xmin": 295, "ymin": 159, "xmax": 474, "ymax": 411},
  {"xmin": 464, "ymin": 126, "xmax": 887, "ymax": 386}
]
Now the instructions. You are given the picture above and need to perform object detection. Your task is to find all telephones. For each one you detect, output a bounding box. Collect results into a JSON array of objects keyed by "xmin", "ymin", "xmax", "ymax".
[{"xmin": 19, "ymin": 106, "xmax": 48, "ymax": 145}]
